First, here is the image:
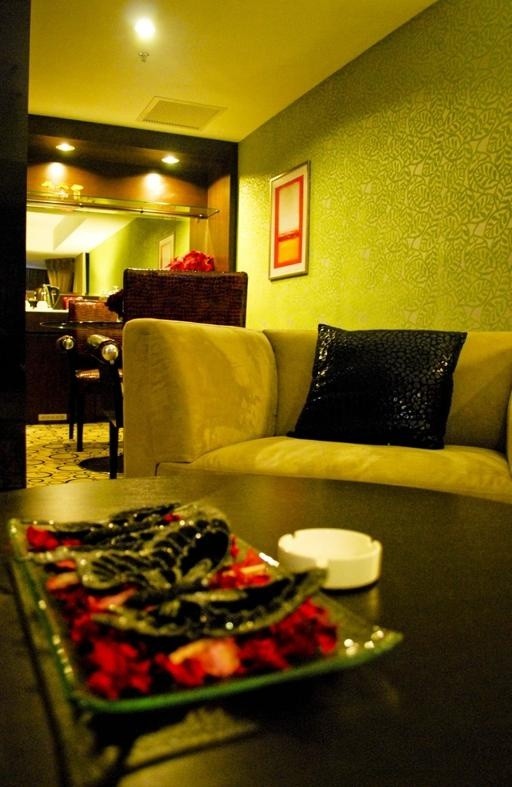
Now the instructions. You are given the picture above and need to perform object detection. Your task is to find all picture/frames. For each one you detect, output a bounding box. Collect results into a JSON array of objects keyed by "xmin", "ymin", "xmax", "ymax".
[{"xmin": 267, "ymin": 158, "xmax": 312, "ymax": 281}]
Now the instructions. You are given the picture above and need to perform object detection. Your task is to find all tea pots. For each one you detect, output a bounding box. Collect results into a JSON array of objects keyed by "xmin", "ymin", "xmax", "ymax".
[{"xmin": 35, "ymin": 283, "xmax": 61, "ymax": 308}]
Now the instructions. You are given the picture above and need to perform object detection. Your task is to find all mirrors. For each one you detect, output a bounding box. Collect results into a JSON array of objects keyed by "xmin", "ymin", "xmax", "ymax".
[{"xmin": 26, "ymin": 200, "xmax": 210, "ymax": 311}]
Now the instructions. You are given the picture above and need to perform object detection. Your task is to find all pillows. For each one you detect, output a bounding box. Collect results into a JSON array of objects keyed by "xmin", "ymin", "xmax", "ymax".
[{"xmin": 285, "ymin": 322, "xmax": 467, "ymax": 450}]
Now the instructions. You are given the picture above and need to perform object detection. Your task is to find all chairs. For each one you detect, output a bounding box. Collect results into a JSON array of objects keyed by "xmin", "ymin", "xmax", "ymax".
[
  {"xmin": 120, "ymin": 316, "xmax": 512, "ymax": 504},
  {"xmin": 68, "ymin": 268, "xmax": 249, "ymax": 479}
]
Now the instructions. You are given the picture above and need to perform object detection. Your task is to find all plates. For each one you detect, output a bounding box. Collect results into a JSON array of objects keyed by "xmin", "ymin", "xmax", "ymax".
[{"xmin": 3, "ymin": 498, "xmax": 408, "ymax": 716}]
